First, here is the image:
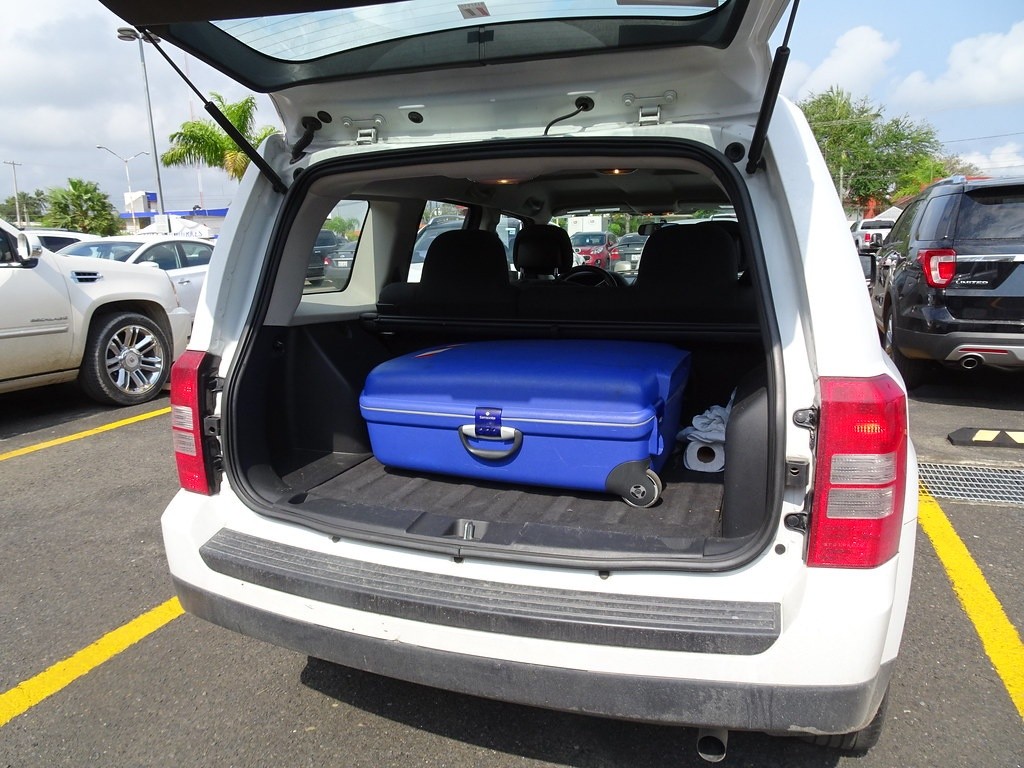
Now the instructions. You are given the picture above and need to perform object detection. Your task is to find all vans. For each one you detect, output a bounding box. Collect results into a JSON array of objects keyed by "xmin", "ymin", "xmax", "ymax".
[
  {"xmin": 304, "ymin": 229, "xmax": 339, "ymax": 286},
  {"xmin": 98, "ymin": 0, "xmax": 920, "ymax": 764},
  {"xmin": 851, "ymin": 218, "xmax": 897, "ymax": 253}
]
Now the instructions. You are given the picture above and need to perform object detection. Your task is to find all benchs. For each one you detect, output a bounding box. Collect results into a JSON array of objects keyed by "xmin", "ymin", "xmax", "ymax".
[{"xmin": 518, "ymin": 224, "xmax": 764, "ymax": 426}]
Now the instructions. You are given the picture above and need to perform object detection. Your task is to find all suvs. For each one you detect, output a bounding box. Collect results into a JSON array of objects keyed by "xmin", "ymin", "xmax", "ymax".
[
  {"xmin": 0, "ymin": 217, "xmax": 193, "ymax": 407},
  {"xmin": 856, "ymin": 175, "xmax": 1024, "ymax": 392}
]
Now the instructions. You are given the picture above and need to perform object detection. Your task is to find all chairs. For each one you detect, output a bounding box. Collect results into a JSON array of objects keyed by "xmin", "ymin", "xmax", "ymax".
[
  {"xmin": 377, "ymin": 229, "xmax": 517, "ymax": 317},
  {"xmin": 700, "ymin": 220, "xmax": 747, "ymax": 272},
  {"xmin": 509, "ymin": 224, "xmax": 573, "ymax": 282}
]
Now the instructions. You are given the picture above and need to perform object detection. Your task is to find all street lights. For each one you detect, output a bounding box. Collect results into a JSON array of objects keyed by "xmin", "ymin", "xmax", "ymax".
[
  {"xmin": 117, "ymin": 26, "xmax": 166, "ymax": 215},
  {"xmin": 96, "ymin": 144, "xmax": 150, "ymax": 234}
]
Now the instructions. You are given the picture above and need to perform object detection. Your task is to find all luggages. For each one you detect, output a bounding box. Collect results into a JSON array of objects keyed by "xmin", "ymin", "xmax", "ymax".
[{"xmin": 359, "ymin": 340, "xmax": 695, "ymax": 508}]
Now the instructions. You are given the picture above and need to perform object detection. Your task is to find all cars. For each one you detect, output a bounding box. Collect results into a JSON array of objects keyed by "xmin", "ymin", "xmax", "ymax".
[
  {"xmin": 324, "ymin": 241, "xmax": 358, "ymax": 289},
  {"xmin": 54, "ymin": 234, "xmax": 216, "ymax": 324},
  {"xmin": 20, "ymin": 229, "xmax": 104, "ymax": 257},
  {"xmin": 407, "ymin": 212, "xmax": 738, "ymax": 285}
]
